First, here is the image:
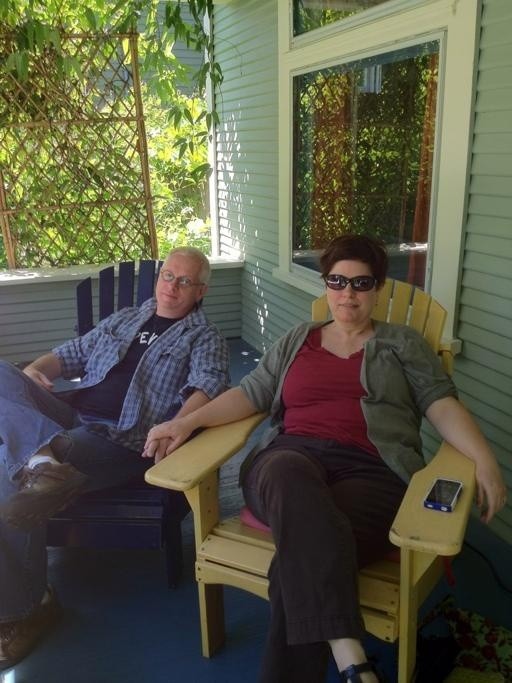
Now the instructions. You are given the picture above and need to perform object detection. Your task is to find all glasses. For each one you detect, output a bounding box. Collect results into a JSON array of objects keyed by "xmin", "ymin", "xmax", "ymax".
[
  {"xmin": 160, "ymin": 270, "xmax": 205, "ymax": 289},
  {"xmin": 323, "ymin": 274, "xmax": 379, "ymax": 292}
]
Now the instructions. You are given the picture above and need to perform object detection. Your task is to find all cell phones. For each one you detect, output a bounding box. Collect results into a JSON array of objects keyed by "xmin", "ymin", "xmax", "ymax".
[{"xmin": 424, "ymin": 477, "xmax": 462, "ymax": 512}]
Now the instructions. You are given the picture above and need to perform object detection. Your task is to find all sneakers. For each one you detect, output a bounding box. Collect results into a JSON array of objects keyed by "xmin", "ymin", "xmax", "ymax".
[
  {"xmin": 0, "ymin": 597, "xmax": 64, "ymax": 672},
  {"xmin": 1, "ymin": 462, "xmax": 90, "ymax": 533}
]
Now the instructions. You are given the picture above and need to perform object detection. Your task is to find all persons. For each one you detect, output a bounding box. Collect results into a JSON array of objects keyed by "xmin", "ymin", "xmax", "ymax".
[
  {"xmin": 143, "ymin": 233, "xmax": 506, "ymax": 682},
  {"xmin": 0, "ymin": 246, "xmax": 232, "ymax": 672}
]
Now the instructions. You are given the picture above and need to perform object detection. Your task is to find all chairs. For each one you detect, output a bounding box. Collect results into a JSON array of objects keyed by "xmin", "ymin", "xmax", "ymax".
[
  {"xmin": 138, "ymin": 275, "xmax": 489, "ymax": 680},
  {"xmin": 13, "ymin": 256, "xmax": 210, "ymax": 581}
]
